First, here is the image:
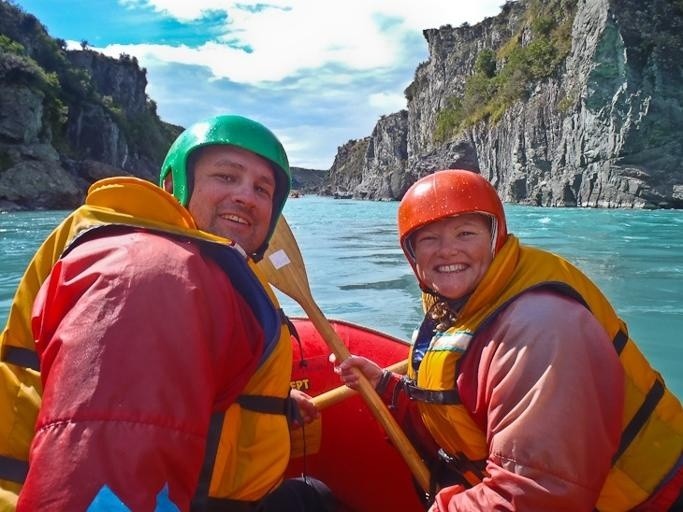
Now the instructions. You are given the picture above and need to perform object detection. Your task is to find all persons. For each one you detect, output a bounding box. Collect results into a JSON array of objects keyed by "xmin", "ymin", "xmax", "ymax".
[
  {"xmin": 0, "ymin": 113, "xmax": 351, "ymax": 512},
  {"xmin": 329, "ymin": 167, "xmax": 683, "ymax": 512}
]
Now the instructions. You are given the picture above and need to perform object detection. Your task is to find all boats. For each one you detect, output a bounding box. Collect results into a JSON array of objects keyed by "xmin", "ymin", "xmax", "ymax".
[{"xmin": 284, "ymin": 311, "xmax": 440, "ymax": 512}]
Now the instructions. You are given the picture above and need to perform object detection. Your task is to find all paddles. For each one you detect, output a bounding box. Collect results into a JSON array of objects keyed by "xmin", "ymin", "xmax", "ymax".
[{"xmin": 255, "ymin": 214, "xmax": 442, "ymax": 493}]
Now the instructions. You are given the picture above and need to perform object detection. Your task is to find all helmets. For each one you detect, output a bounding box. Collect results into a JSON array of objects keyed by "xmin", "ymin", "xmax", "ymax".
[
  {"xmin": 398, "ymin": 170, "xmax": 507, "ymax": 294},
  {"xmin": 159, "ymin": 115, "xmax": 292, "ymax": 263}
]
